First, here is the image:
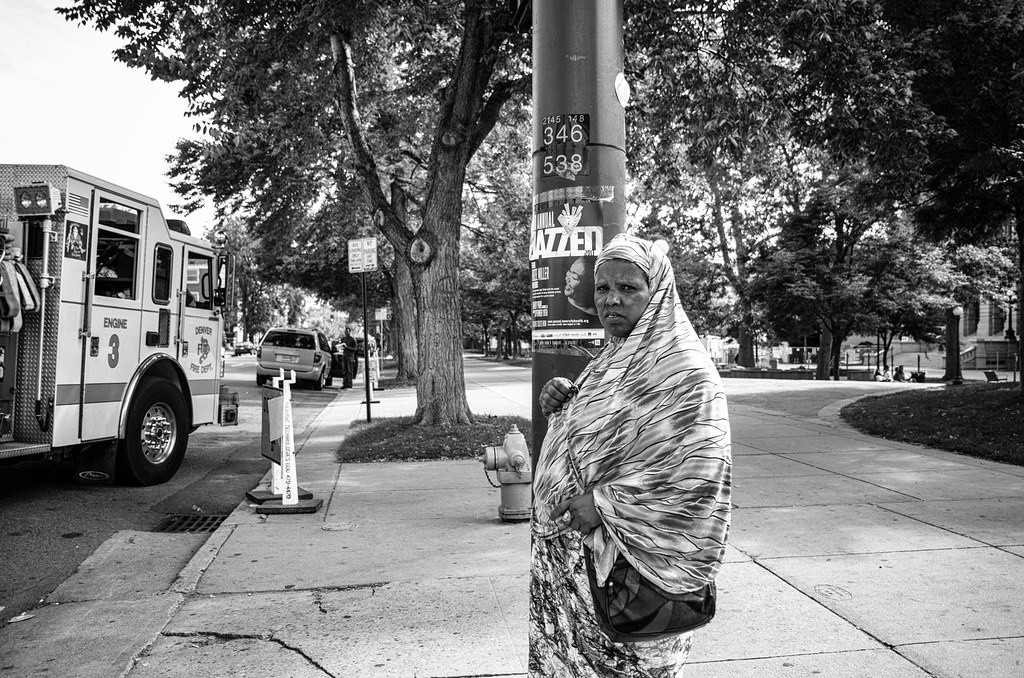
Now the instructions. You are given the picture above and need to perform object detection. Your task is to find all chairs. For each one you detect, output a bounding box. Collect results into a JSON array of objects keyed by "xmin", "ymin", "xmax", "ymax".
[{"xmin": 984, "ymin": 371, "xmax": 1008, "ymax": 383}]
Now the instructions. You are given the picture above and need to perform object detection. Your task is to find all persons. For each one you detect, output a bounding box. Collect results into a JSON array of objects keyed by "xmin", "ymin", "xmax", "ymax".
[
  {"xmin": 874, "ymin": 365, "xmax": 905, "ymax": 382},
  {"xmin": 528, "ymin": 233, "xmax": 732, "ymax": 678},
  {"xmin": 564, "ymin": 257, "xmax": 597, "ymax": 316},
  {"xmin": 340, "ymin": 328, "xmax": 357, "ymax": 389}
]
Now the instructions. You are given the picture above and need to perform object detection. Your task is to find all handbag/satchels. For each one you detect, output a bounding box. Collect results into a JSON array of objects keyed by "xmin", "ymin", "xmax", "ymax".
[{"xmin": 583, "ymin": 542, "xmax": 716, "ymax": 642}]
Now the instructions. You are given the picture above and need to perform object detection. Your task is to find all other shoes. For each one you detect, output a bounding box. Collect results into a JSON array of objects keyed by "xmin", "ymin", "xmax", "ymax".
[{"xmin": 339, "ymin": 385, "xmax": 352, "ymax": 389}]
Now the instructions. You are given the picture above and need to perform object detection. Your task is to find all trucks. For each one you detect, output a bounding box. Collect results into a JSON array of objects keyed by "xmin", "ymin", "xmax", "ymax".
[{"xmin": 0, "ymin": 163, "xmax": 241, "ymax": 486}]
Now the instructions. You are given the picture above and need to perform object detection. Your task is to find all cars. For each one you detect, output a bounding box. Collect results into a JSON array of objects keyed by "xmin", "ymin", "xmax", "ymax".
[{"xmin": 256, "ymin": 326, "xmax": 358, "ymax": 391}]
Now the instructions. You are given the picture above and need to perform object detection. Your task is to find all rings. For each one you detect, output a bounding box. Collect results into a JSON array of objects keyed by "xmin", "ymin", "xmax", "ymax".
[{"xmin": 561, "ymin": 510, "xmax": 572, "ymax": 522}]
